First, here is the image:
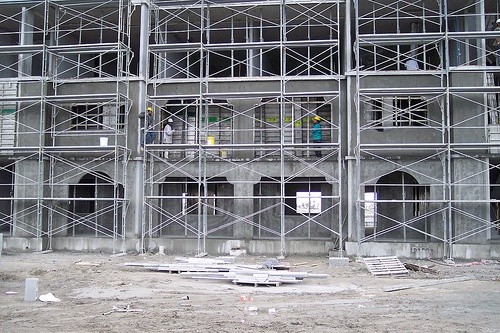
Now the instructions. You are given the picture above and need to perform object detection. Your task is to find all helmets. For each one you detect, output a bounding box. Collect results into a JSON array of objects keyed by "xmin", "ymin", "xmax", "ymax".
[
  {"xmin": 146, "ymin": 107, "xmax": 152, "ymax": 111},
  {"xmin": 313, "ymin": 116, "xmax": 320, "ymax": 121},
  {"xmin": 168, "ymin": 118, "xmax": 173, "ymax": 123},
  {"xmin": 495, "ymin": 19, "xmax": 500, "ymax": 23}
]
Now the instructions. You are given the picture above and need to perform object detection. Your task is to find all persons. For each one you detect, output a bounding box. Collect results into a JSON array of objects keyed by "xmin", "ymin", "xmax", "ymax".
[
  {"xmin": 309, "ymin": 116, "xmax": 322, "ymax": 157},
  {"xmin": 494, "ymin": 19, "xmax": 500, "ymax": 66},
  {"xmin": 405, "ymin": 58, "xmax": 419, "ymax": 71},
  {"xmin": 138, "ymin": 107, "xmax": 156, "ymax": 147},
  {"xmin": 163, "ymin": 118, "xmax": 176, "ymax": 159}
]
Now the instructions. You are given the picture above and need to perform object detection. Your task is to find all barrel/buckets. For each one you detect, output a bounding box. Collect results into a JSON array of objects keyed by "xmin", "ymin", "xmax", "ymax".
[
  {"xmin": 100, "ymin": 137, "xmax": 108, "ymax": 146},
  {"xmin": 219, "ymin": 150, "xmax": 228, "ymax": 159},
  {"xmin": 207, "ymin": 136, "xmax": 214, "ymax": 145}
]
